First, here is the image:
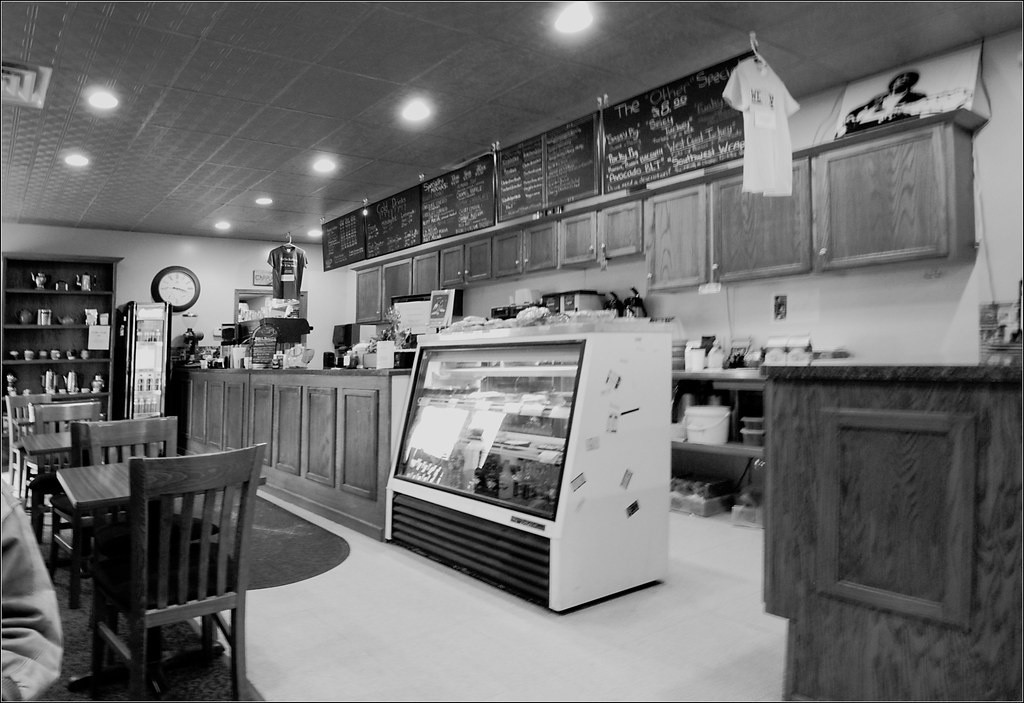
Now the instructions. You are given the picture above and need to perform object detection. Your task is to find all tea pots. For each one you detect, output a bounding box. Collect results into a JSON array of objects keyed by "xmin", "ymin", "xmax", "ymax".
[
  {"xmin": 30, "ymin": 270, "xmax": 47, "ymax": 288},
  {"xmin": 63, "ymin": 370, "xmax": 79, "ymax": 395},
  {"xmin": 57, "ymin": 314, "xmax": 77, "ymax": 324},
  {"xmin": 40, "ymin": 368, "xmax": 56, "ymax": 395},
  {"xmin": 75, "ymin": 272, "xmax": 96, "ymax": 291},
  {"xmin": 14, "ymin": 307, "xmax": 37, "ymax": 324}
]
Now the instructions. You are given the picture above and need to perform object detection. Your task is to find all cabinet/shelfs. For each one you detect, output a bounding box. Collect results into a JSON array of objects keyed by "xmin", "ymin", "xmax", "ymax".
[
  {"xmin": 350, "ymin": 107, "xmax": 990, "ymax": 325},
  {"xmin": 0, "ymin": 251, "xmax": 125, "ymax": 473},
  {"xmin": 168, "ymin": 331, "xmax": 1024, "ymax": 703}
]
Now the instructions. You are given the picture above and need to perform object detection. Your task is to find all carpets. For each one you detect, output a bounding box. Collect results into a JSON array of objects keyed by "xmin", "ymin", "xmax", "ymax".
[
  {"xmin": 30, "ymin": 514, "xmax": 264, "ymax": 703},
  {"xmin": 174, "ymin": 487, "xmax": 351, "ymax": 590}
]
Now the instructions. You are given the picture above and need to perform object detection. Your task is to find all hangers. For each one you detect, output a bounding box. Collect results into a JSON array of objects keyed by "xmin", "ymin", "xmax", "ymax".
[
  {"xmin": 271, "ymin": 235, "xmax": 306, "ymax": 254},
  {"xmin": 737, "ymin": 31, "xmax": 780, "ymax": 81}
]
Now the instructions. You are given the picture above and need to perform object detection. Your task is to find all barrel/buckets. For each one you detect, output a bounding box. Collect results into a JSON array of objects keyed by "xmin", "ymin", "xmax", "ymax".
[{"xmin": 682, "ymin": 405, "xmax": 729, "ymax": 444}]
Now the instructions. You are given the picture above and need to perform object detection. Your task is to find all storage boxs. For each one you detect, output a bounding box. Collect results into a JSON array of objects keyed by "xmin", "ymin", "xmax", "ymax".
[
  {"xmin": 740, "ymin": 427, "xmax": 765, "ymax": 446},
  {"xmin": 740, "ymin": 417, "xmax": 765, "ymax": 429},
  {"xmin": 364, "ymin": 354, "xmax": 377, "ymax": 368},
  {"xmin": 560, "ymin": 289, "xmax": 608, "ymax": 313},
  {"xmin": 332, "ymin": 324, "xmax": 360, "ymax": 349},
  {"xmin": 220, "ymin": 344, "xmax": 251, "ymax": 368},
  {"xmin": 681, "ymin": 405, "xmax": 731, "ymax": 445},
  {"xmin": 542, "ymin": 294, "xmax": 560, "ymax": 313}
]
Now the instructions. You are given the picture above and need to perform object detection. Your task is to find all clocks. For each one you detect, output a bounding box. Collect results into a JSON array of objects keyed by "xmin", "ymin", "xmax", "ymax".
[{"xmin": 151, "ymin": 266, "xmax": 200, "ymax": 312}]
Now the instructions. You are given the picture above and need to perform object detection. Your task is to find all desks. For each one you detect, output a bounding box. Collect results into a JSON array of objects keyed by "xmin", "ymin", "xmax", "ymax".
[
  {"xmin": 56, "ymin": 462, "xmax": 130, "ymax": 510},
  {"xmin": 20, "ymin": 432, "xmax": 71, "ymax": 456},
  {"xmin": 12, "ymin": 418, "xmax": 34, "ymax": 427}
]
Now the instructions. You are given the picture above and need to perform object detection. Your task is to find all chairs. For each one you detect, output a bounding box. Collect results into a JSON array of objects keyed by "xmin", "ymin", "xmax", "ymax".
[
  {"xmin": 28, "ymin": 402, "xmax": 101, "ymax": 543},
  {"xmin": 5, "ymin": 394, "xmax": 52, "ymax": 498},
  {"xmin": 90, "ymin": 416, "xmax": 178, "ymax": 628},
  {"xmin": 49, "ymin": 422, "xmax": 90, "ymax": 610},
  {"xmin": 93, "ymin": 441, "xmax": 268, "ymax": 702}
]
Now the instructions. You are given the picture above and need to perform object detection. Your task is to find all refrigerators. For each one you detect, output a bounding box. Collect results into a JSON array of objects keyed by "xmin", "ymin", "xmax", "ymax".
[{"xmin": 113, "ymin": 300, "xmax": 172, "ymax": 456}]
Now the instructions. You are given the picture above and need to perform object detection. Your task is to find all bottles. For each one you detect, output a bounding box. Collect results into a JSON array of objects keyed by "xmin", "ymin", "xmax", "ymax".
[
  {"xmin": 135, "ymin": 328, "xmax": 162, "ymax": 412},
  {"xmin": 498, "ymin": 457, "xmax": 560, "ymax": 506}
]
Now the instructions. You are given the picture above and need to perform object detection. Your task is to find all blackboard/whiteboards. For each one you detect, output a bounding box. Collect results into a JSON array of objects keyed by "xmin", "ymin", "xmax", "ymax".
[
  {"xmin": 366, "ymin": 184, "xmax": 421, "ymax": 260},
  {"xmin": 321, "ymin": 206, "xmax": 367, "ymax": 272},
  {"xmin": 601, "ymin": 50, "xmax": 755, "ymax": 195},
  {"xmin": 421, "ymin": 153, "xmax": 495, "ymax": 244},
  {"xmin": 497, "ymin": 132, "xmax": 546, "ymax": 222},
  {"xmin": 543, "ymin": 110, "xmax": 600, "ymax": 211}
]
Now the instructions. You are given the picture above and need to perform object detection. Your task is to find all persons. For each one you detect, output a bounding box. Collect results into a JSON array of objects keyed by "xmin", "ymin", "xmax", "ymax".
[{"xmin": 1, "ymin": 479, "xmax": 65, "ymax": 701}]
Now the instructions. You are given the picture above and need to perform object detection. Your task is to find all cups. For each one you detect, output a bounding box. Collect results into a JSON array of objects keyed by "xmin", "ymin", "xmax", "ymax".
[
  {"xmin": 24, "ymin": 350, "xmax": 61, "ymax": 361},
  {"xmin": 684, "ymin": 348, "xmax": 705, "ymax": 373},
  {"xmin": 81, "ymin": 388, "xmax": 91, "ymax": 393},
  {"xmin": 323, "ymin": 352, "xmax": 336, "ymax": 369},
  {"xmin": 59, "ymin": 388, "xmax": 66, "ymax": 394}
]
